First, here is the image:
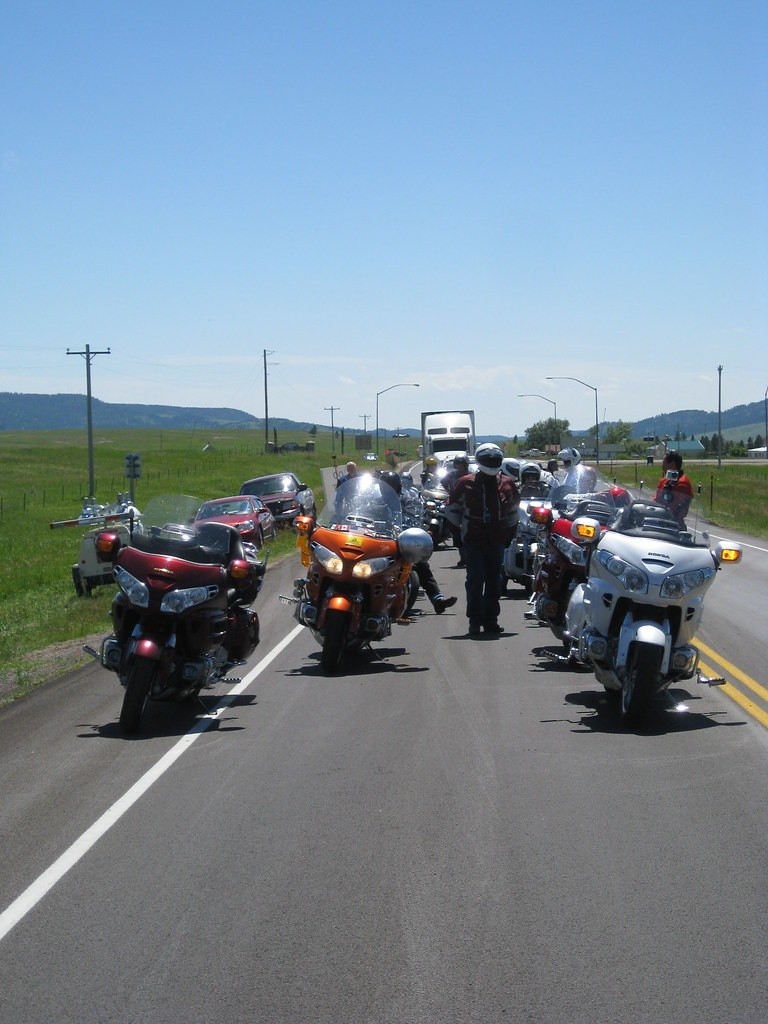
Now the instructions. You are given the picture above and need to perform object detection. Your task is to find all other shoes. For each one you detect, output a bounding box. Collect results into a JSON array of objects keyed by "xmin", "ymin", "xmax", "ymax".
[
  {"xmin": 469, "ymin": 625, "xmax": 481, "ymax": 637},
  {"xmin": 434, "ymin": 596, "xmax": 457, "ymax": 614},
  {"xmin": 458, "ymin": 560, "xmax": 465, "ymax": 567},
  {"xmin": 484, "ymin": 621, "xmax": 504, "ymax": 637}
]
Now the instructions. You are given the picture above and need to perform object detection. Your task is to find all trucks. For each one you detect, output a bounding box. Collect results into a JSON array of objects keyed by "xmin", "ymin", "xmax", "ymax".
[{"xmin": 420, "ymin": 410, "xmax": 476, "ymax": 469}]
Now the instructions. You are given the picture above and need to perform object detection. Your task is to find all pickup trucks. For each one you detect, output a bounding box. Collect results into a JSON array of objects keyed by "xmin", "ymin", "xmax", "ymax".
[{"xmin": 518, "ymin": 449, "xmax": 547, "ymax": 459}]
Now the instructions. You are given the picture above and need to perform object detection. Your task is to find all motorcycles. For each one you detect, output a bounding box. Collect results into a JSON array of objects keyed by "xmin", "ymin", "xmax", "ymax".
[
  {"xmin": 530, "ymin": 479, "xmax": 643, "ymax": 668},
  {"xmin": 539, "ymin": 489, "xmax": 743, "ymax": 724},
  {"xmin": 399, "ymin": 468, "xmax": 560, "ymax": 596},
  {"xmin": 278, "ymin": 475, "xmax": 434, "ymax": 671},
  {"xmin": 80, "ymin": 494, "xmax": 270, "ymax": 735}
]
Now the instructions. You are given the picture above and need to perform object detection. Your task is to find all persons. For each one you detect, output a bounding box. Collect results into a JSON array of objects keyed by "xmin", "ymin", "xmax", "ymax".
[
  {"xmin": 653, "ymin": 450, "xmax": 692, "ymax": 531},
  {"xmin": 452, "ymin": 442, "xmax": 521, "ymax": 635},
  {"xmin": 500, "ymin": 446, "xmax": 598, "ymax": 595},
  {"xmin": 336, "ymin": 454, "xmax": 474, "ymax": 617}
]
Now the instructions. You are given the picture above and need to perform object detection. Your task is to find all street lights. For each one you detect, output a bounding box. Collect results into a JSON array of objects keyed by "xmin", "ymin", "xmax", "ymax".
[
  {"xmin": 376, "ymin": 383, "xmax": 420, "ymax": 456},
  {"xmin": 546, "ymin": 377, "xmax": 599, "ymax": 466},
  {"xmin": 518, "ymin": 393, "xmax": 558, "ymax": 456}
]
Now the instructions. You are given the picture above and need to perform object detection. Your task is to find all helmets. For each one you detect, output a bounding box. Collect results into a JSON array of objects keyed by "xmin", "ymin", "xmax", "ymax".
[
  {"xmin": 425, "ymin": 458, "xmax": 437, "ymax": 465},
  {"xmin": 401, "ymin": 472, "xmax": 413, "ymax": 480},
  {"xmin": 502, "ymin": 458, "xmax": 520, "ymax": 481},
  {"xmin": 380, "ymin": 472, "xmax": 402, "ymax": 491},
  {"xmin": 521, "ymin": 463, "xmax": 541, "ymax": 482},
  {"xmin": 475, "ymin": 443, "xmax": 503, "ymax": 477},
  {"xmin": 453, "ymin": 454, "xmax": 469, "ymax": 469},
  {"xmin": 557, "ymin": 447, "xmax": 582, "ymax": 466}
]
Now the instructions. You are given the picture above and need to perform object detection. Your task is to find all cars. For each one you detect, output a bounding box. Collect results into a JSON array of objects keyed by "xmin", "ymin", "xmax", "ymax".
[
  {"xmin": 393, "ymin": 433, "xmax": 410, "ymax": 438},
  {"xmin": 440, "ymin": 455, "xmax": 478, "ymax": 473},
  {"xmin": 191, "ymin": 495, "xmax": 276, "ymax": 551},
  {"xmin": 238, "ymin": 472, "xmax": 317, "ymax": 530}
]
